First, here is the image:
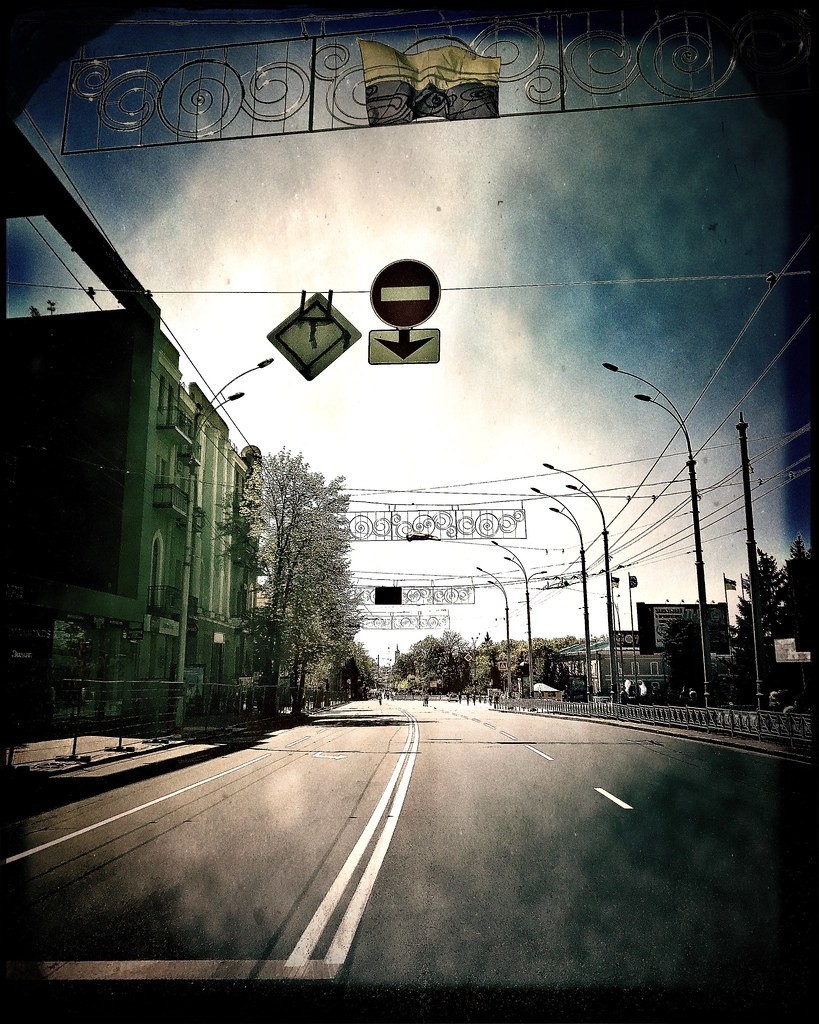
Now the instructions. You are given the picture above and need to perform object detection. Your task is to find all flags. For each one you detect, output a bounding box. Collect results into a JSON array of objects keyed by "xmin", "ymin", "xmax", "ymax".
[
  {"xmin": 612, "ymin": 576, "xmax": 637, "ymax": 587},
  {"xmin": 725, "ymin": 577, "xmax": 750, "ymax": 590}
]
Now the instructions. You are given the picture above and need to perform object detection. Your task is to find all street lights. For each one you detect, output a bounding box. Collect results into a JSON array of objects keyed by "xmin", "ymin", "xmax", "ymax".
[
  {"xmin": 531, "ymin": 486, "xmax": 594, "ymax": 701},
  {"xmin": 542, "ymin": 460, "xmax": 619, "ymax": 701},
  {"xmin": 176, "ymin": 357, "xmax": 275, "ymax": 728},
  {"xmin": 490, "ymin": 538, "xmax": 547, "ymax": 700},
  {"xmin": 475, "ymin": 565, "xmax": 511, "ymax": 698},
  {"xmin": 603, "ymin": 359, "xmax": 715, "ymax": 713}
]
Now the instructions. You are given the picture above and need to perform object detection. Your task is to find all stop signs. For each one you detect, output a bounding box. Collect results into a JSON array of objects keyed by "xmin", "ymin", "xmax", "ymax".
[{"xmin": 371, "ymin": 258, "xmax": 440, "ymax": 328}]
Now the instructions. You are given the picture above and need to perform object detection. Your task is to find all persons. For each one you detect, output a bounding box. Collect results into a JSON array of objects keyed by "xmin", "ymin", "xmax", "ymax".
[
  {"xmin": 597, "ymin": 688, "xmax": 627, "ymax": 705},
  {"xmin": 378, "ymin": 692, "xmax": 544, "ymax": 707},
  {"xmin": 679, "ymin": 685, "xmax": 697, "ymax": 706},
  {"xmin": 770, "ymin": 689, "xmax": 792, "ymax": 710}
]
